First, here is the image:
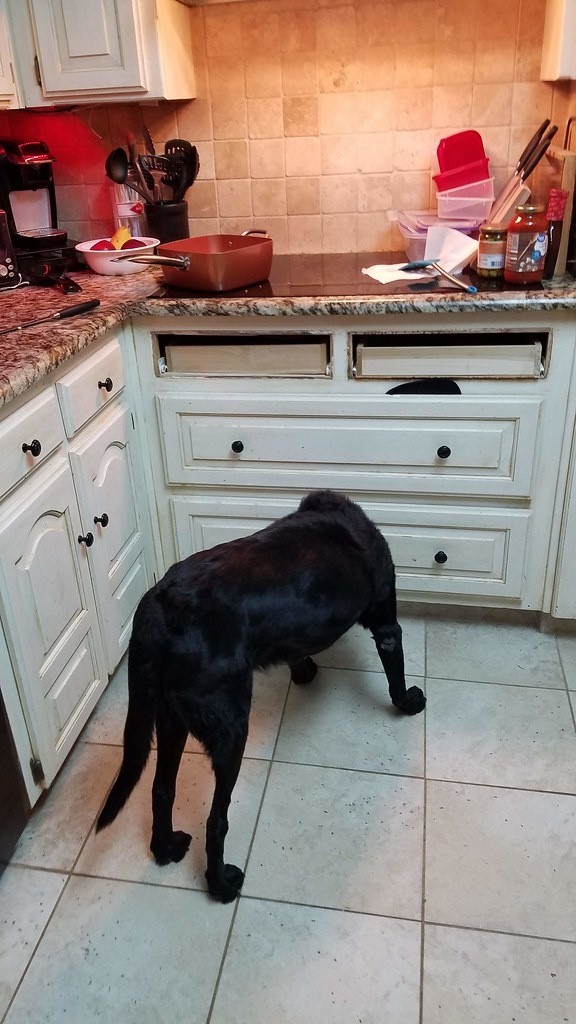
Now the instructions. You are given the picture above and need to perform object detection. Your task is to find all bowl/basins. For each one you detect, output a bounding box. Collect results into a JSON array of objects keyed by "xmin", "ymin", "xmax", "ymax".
[{"xmin": 72, "ymin": 235, "xmax": 162, "ymax": 277}]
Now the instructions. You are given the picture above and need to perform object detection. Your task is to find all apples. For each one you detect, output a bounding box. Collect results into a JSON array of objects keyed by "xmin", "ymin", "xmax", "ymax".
[
  {"xmin": 121, "ymin": 239, "xmax": 148, "ymax": 250},
  {"xmin": 89, "ymin": 240, "xmax": 116, "ymax": 250}
]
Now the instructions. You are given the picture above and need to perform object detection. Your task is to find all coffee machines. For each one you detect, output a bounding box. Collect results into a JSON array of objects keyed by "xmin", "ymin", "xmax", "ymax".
[{"xmin": 0, "ymin": 137, "xmax": 77, "ymax": 278}]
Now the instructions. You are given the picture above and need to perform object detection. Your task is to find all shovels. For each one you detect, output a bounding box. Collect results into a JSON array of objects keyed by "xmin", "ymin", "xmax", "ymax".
[
  {"xmin": 400, "ymin": 257, "xmax": 478, "ymax": 295},
  {"xmin": 139, "ymin": 138, "xmax": 192, "ymax": 206}
]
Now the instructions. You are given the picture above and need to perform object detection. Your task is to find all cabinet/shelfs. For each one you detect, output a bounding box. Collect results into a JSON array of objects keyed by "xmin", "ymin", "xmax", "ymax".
[
  {"xmin": 0, "ymin": 0, "xmax": 26, "ymax": 112},
  {"xmin": 27, "ymin": 1, "xmax": 199, "ymax": 109},
  {"xmin": 0, "ymin": 325, "xmax": 160, "ymax": 813},
  {"xmin": 126, "ymin": 310, "xmax": 575, "ymax": 611},
  {"xmin": 551, "ymin": 417, "xmax": 574, "ymax": 620}
]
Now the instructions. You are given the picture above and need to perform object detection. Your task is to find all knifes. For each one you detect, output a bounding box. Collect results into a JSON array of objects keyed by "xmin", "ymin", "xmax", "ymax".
[{"xmin": 509, "ymin": 118, "xmax": 560, "ymax": 185}]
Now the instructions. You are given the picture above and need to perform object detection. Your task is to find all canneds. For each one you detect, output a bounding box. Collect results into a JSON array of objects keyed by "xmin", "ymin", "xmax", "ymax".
[
  {"xmin": 504, "ymin": 203, "xmax": 548, "ymax": 285},
  {"xmin": 477, "ymin": 223, "xmax": 507, "ymax": 279}
]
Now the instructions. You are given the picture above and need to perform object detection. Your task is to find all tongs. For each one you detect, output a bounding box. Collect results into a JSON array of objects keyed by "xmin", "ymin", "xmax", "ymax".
[{"xmin": 119, "ymin": 124, "xmax": 163, "ymax": 202}]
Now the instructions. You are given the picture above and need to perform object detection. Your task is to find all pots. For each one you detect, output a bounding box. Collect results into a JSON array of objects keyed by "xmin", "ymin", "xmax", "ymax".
[{"xmin": 109, "ymin": 228, "xmax": 275, "ymax": 291}]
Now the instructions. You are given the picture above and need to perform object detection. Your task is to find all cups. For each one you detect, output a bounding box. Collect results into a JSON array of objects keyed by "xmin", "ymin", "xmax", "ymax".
[{"xmin": 144, "ymin": 200, "xmax": 190, "ymax": 244}]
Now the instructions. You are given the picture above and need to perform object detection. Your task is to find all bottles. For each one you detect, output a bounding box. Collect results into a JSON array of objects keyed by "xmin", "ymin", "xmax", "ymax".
[{"xmin": 541, "ymin": 187, "xmax": 570, "ymax": 280}]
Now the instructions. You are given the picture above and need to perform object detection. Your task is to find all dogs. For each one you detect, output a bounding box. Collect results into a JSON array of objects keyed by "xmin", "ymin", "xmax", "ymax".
[{"xmin": 93, "ymin": 490, "xmax": 426, "ymax": 904}]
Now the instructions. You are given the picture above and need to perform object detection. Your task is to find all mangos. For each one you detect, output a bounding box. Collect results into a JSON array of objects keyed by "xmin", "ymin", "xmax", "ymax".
[{"xmin": 109, "ymin": 226, "xmax": 130, "ymax": 251}]
